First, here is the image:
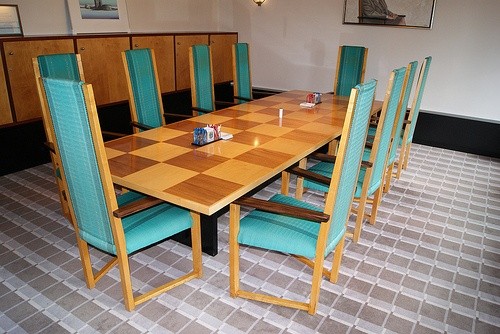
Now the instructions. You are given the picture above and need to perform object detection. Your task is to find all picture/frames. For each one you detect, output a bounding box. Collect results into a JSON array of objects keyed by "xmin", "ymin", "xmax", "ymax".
[
  {"xmin": 66, "ymin": 0, "xmax": 131, "ymax": 36},
  {"xmin": 341, "ymin": 0, "xmax": 437, "ymax": 30}
]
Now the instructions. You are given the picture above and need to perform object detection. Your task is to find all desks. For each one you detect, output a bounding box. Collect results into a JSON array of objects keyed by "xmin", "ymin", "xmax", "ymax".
[{"xmin": 103, "ymin": 88, "xmax": 385, "ymax": 258}]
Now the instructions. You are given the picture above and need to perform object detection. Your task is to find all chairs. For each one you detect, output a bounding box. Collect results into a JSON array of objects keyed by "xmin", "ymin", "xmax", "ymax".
[{"xmin": 31, "ymin": 43, "xmax": 433, "ymax": 316}]
